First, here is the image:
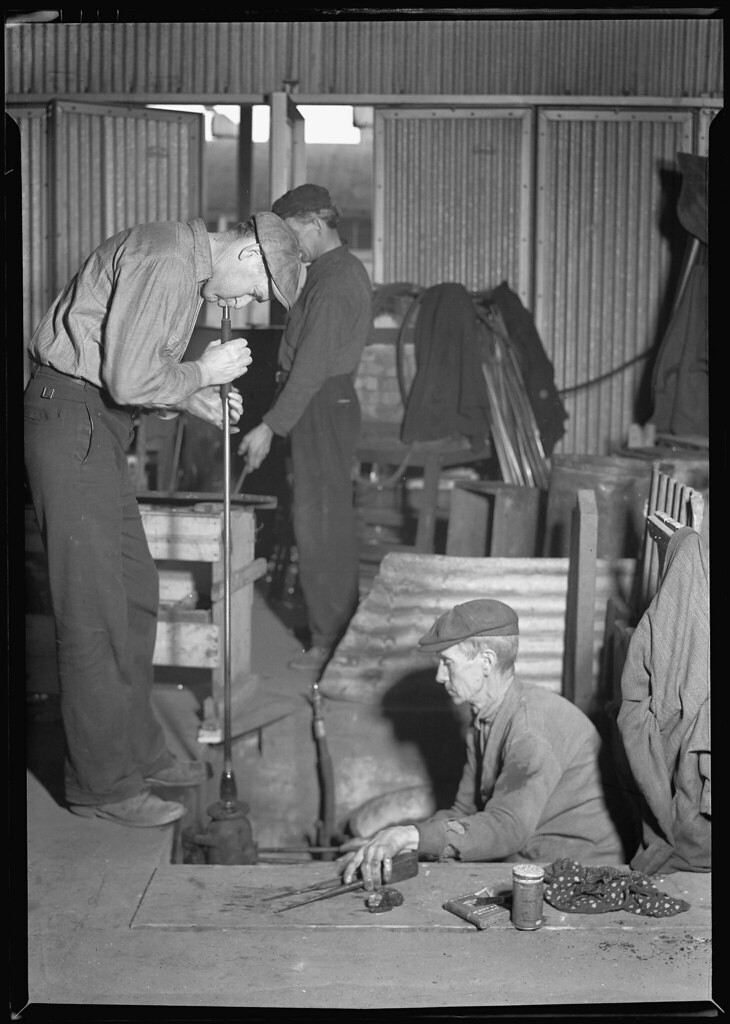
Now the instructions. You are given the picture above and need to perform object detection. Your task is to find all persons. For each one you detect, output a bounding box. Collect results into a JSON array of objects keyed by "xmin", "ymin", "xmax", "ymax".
[
  {"xmin": 339, "ymin": 599, "xmax": 624, "ymax": 893},
  {"xmin": 20, "ymin": 211, "xmax": 304, "ymax": 829},
  {"xmin": 236, "ymin": 183, "xmax": 373, "ymax": 670}
]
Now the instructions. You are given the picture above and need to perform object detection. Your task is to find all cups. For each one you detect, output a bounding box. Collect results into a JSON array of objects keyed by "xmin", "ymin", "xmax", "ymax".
[{"xmin": 512, "ymin": 864, "xmax": 545, "ymax": 931}]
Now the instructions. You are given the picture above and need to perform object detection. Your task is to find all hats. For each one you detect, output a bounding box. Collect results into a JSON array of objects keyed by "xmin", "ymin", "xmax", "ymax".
[
  {"xmin": 417, "ymin": 599, "xmax": 519, "ymax": 653},
  {"xmin": 250, "ymin": 210, "xmax": 302, "ymax": 311},
  {"xmin": 271, "ymin": 183, "xmax": 331, "ymax": 221}
]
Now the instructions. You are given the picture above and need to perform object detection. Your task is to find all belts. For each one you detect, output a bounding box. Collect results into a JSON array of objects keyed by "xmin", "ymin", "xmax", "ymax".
[{"xmin": 34, "ymin": 363, "xmax": 108, "ymax": 396}]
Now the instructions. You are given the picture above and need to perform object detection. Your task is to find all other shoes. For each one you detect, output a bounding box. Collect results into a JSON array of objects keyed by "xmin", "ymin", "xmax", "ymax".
[
  {"xmin": 69, "ymin": 788, "xmax": 187, "ymax": 827},
  {"xmin": 289, "ymin": 648, "xmax": 333, "ymax": 669},
  {"xmin": 141, "ymin": 758, "xmax": 213, "ymax": 789}
]
